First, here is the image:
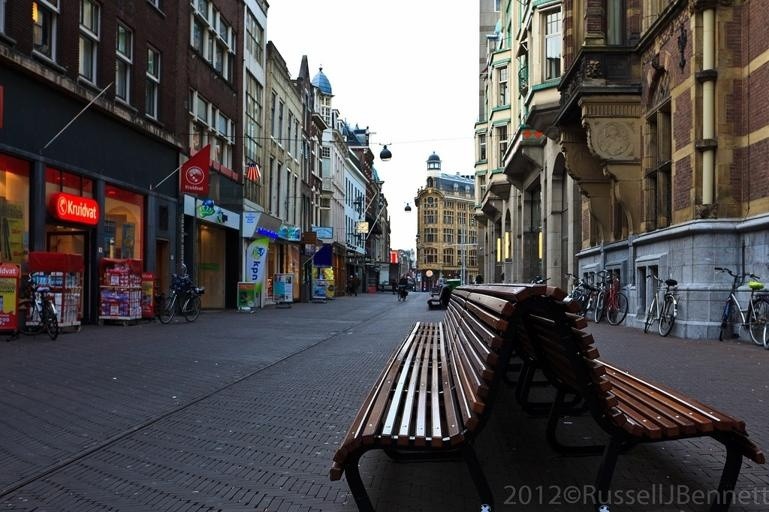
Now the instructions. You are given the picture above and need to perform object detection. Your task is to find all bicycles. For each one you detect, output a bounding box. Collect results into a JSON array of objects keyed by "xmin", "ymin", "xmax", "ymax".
[
  {"xmin": 562, "ymin": 269, "xmax": 629, "ymax": 326},
  {"xmin": 643, "ymin": 273, "xmax": 680, "ymax": 337},
  {"xmin": 157, "ymin": 272, "xmax": 206, "ymax": 324},
  {"xmin": 528, "ymin": 275, "xmax": 550, "ymax": 284},
  {"xmin": 397, "ymin": 286, "xmax": 406, "ymax": 303},
  {"xmin": 19, "ymin": 270, "xmax": 59, "ymax": 340},
  {"xmin": 762, "ymin": 322, "xmax": 769, "ymax": 350},
  {"xmin": 714, "ymin": 267, "xmax": 769, "ymax": 346}
]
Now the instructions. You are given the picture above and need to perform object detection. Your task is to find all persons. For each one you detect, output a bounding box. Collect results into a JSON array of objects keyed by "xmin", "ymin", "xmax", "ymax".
[
  {"xmin": 398, "ymin": 274, "xmax": 408, "ymax": 292},
  {"xmin": 346, "ymin": 274, "xmax": 361, "ymax": 297},
  {"xmin": 390, "ymin": 279, "xmax": 398, "ymax": 295},
  {"xmin": 475, "ymin": 271, "xmax": 483, "ymax": 284}
]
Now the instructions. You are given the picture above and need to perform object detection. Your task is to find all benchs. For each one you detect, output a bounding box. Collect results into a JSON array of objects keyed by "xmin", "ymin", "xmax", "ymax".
[{"xmin": 329, "ymin": 283, "xmax": 765, "ymax": 511}]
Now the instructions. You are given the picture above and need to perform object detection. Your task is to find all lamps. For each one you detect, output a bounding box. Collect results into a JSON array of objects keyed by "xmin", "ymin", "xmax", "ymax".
[
  {"xmin": 380, "ymin": 144, "xmax": 393, "ymax": 162},
  {"xmin": 404, "ymin": 202, "xmax": 411, "ymax": 211}
]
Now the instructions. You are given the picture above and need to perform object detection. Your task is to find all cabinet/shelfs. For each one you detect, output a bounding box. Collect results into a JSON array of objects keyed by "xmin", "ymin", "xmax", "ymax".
[
  {"xmin": 25, "ymin": 252, "xmax": 85, "ymax": 333},
  {"xmin": 98, "ymin": 256, "xmax": 144, "ymax": 327}
]
